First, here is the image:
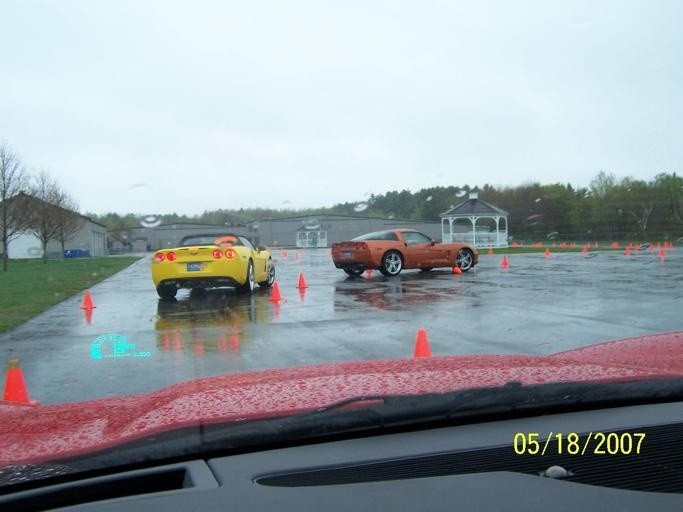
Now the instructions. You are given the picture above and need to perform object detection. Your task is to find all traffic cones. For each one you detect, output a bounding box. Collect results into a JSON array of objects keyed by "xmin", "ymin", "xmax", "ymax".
[
  {"xmin": 509, "ymin": 236, "xmax": 678, "ymax": 257},
  {"xmin": 367, "ymin": 268, "xmax": 373, "ymax": 273},
  {"xmin": 78, "ymin": 289, "xmax": 98, "ymax": 310},
  {"xmin": 265, "ymin": 280, "xmax": 285, "ymax": 303},
  {"xmin": 1, "ymin": 356, "xmax": 41, "ymax": 407},
  {"xmin": 501, "ymin": 254, "xmax": 508, "ymax": 266},
  {"xmin": 82, "ymin": 308, "xmax": 93, "ymax": 325},
  {"xmin": 294, "ymin": 272, "xmax": 310, "ymax": 289},
  {"xmin": 486, "ymin": 246, "xmax": 493, "ymax": 255},
  {"xmin": 411, "ymin": 327, "xmax": 433, "ymax": 359},
  {"xmin": 298, "ymin": 286, "xmax": 307, "ymax": 301},
  {"xmin": 452, "ymin": 264, "xmax": 462, "ymax": 275},
  {"xmin": 279, "ymin": 245, "xmax": 307, "ymax": 261},
  {"xmin": 272, "ymin": 298, "xmax": 282, "ymax": 321}
]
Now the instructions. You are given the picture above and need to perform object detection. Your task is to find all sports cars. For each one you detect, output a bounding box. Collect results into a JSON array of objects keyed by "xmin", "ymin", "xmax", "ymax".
[
  {"xmin": 154, "ymin": 285, "xmax": 275, "ymax": 356},
  {"xmin": 329, "ymin": 227, "xmax": 484, "ymax": 278},
  {"xmin": 332, "ymin": 272, "xmax": 467, "ymax": 315},
  {"xmin": 148, "ymin": 230, "xmax": 277, "ymax": 302}
]
